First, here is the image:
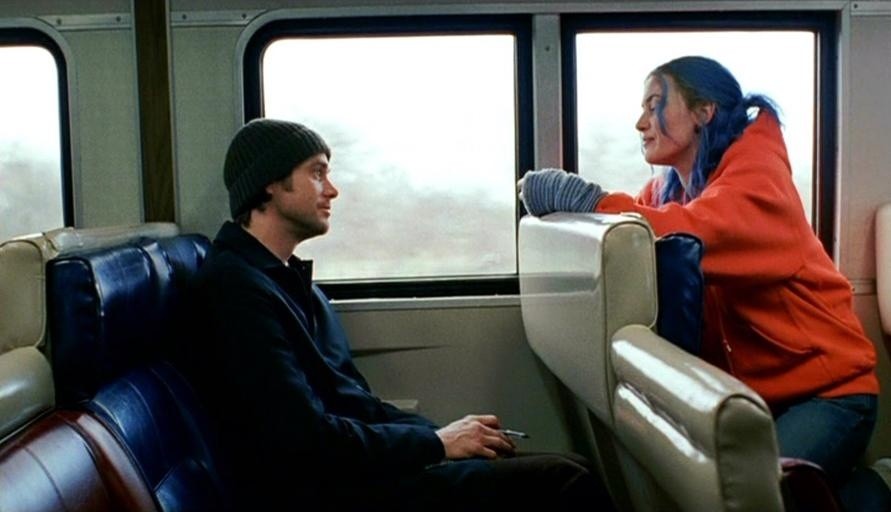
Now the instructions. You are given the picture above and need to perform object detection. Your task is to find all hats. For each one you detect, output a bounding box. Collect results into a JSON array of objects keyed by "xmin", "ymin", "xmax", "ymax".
[{"xmin": 224, "ymin": 118, "xmax": 331, "ymax": 218}]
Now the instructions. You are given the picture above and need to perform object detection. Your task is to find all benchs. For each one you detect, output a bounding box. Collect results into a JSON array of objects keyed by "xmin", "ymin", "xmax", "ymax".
[{"xmin": 515, "ymin": 211, "xmax": 785, "ymax": 512}]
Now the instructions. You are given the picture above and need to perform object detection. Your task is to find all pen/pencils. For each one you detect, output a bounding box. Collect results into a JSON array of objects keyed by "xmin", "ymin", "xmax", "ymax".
[{"xmin": 498, "ymin": 429, "xmax": 530, "ymax": 438}]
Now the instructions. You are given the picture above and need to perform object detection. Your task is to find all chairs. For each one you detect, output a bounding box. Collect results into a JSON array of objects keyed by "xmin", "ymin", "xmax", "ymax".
[{"xmin": 1, "ymin": 222, "xmax": 417, "ymax": 511}]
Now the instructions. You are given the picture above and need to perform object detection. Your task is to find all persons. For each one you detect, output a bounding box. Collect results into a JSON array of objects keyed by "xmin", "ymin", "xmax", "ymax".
[
  {"xmin": 173, "ymin": 116, "xmax": 618, "ymax": 512},
  {"xmin": 516, "ymin": 56, "xmax": 890, "ymax": 512}
]
